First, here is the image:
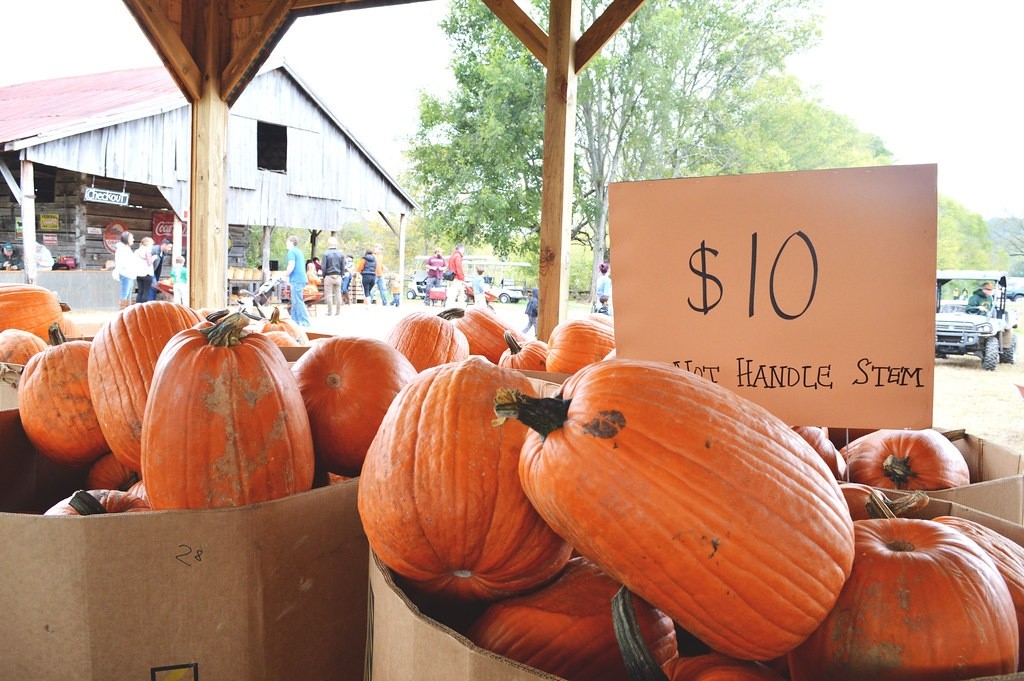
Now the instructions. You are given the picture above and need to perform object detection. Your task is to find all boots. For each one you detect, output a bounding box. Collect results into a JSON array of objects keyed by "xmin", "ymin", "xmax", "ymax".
[{"xmin": 120, "ymin": 300, "xmax": 130, "ymax": 310}]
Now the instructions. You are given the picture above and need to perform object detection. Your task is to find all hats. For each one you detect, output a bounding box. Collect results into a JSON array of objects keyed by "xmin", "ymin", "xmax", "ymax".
[{"xmin": 4, "ymin": 242, "xmax": 14, "ymax": 251}]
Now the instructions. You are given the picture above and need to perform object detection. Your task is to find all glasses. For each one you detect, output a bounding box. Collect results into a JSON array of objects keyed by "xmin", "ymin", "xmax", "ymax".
[{"xmin": 985, "ymin": 288, "xmax": 992, "ymax": 291}]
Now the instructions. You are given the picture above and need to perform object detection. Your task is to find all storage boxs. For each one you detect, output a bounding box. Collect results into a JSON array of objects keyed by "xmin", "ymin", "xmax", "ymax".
[{"xmin": 0, "ymin": 332, "xmax": 1024, "ymax": 681}]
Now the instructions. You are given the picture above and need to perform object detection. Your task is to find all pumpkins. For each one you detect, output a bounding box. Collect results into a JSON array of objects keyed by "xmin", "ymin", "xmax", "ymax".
[{"xmin": 0, "ymin": 283, "xmax": 1024, "ymax": 681}]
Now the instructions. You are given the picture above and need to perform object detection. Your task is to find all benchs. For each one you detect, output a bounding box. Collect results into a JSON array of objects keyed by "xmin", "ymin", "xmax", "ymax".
[{"xmin": 940, "ymin": 305, "xmax": 997, "ymax": 318}]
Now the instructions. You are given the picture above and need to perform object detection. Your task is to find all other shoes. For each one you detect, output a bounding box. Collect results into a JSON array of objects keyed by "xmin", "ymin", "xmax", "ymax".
[{"xmin": 372, "ymin": 300, "xmax": 376, "ymax": 304}]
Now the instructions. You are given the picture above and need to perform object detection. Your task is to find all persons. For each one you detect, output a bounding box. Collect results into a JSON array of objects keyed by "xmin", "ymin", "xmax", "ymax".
[
  {"xmin": 444, "ymin": 243, "xmax": 466, "ymax": 311},
  {"xmin": 423, "ymin": 248, "xmax": 445, "ymax": 306},
  {"xmin": 115, "ymin": 232, "xmax": 139, "ymax": 311},
  {"xmin": 522, "ymin": 288, "xmax": 539, "ymax": 337},
  {"xmin": 388, "ymin": 276, "xmax": 401, "ymax": 307},
  {"xmin": 320, "ymin": 237, "xmax": 345, "ymax": 316},
  {"xmin": 35, "ymin": 242, "xmax": 54, "ymax": 270},
  {"xmin": 472, "ymin": 267, "xmax": 488, "ymax": 312},
  {"xmin": 595, "ymin": 263, "xmax": 613, "ymax": 316},
  {"xmin": 953, "ymin": 289, "xmax": 968, "ymax": 300},
  {"xmin": 965, "ymin": 282, "xmax": 995, "ymax": 317},
  {"xmin": 236, "ymin": 285, "xmax": 271, "ymax": 305},
  {"xmin": 0, "ymin": 242, "xmax": 25, "ymax": 270},
  {"xmin": 169, "ymin": 256, "xmax": 190, "ymax": 307},
  {"xmin": 277, "ymin": 237, "xmax": 310, "ymax": 327},
  {"xmin": 133, "ymin": 237, "xmax": 158, "ymax": 303},
  {"xmin": 147, "ymin": 237, "xmax": 173, "ymax": 301},
  {"xmin": 304, "ymin": 245, "xmax": 387, "ymax": 306}
]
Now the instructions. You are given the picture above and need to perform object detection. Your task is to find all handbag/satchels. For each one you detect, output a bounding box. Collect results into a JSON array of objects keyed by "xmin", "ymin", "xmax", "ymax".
[{"xmin": 443, "ymin": 270, "xmax": 454, "ymax": 281}]
